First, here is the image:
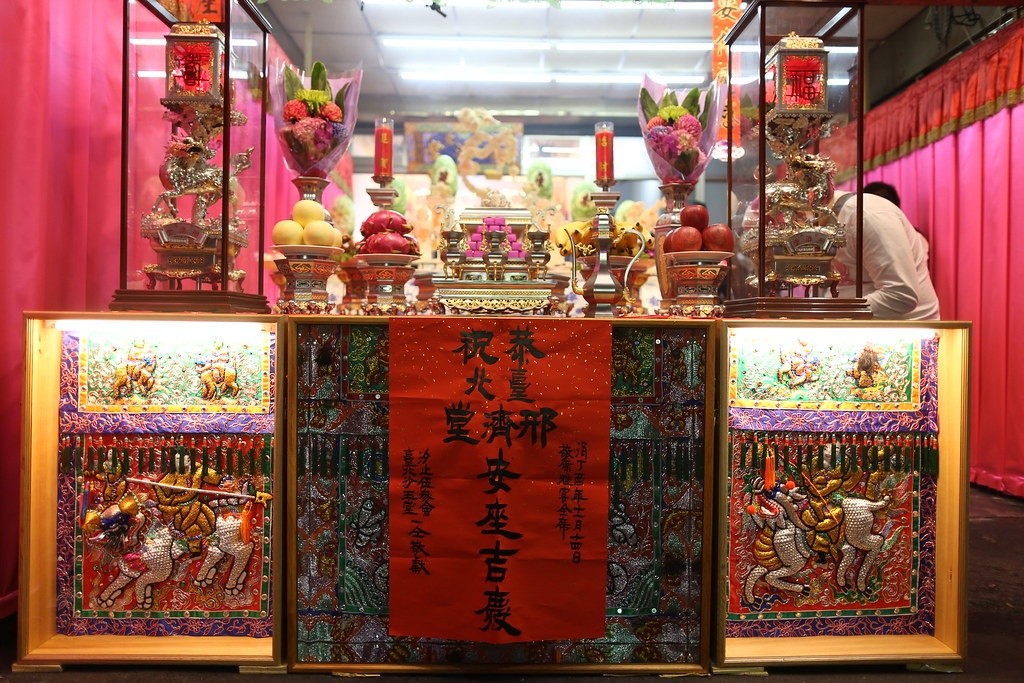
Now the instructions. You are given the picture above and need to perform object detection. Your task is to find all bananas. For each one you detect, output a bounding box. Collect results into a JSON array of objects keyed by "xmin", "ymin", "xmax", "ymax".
[{"xmin": 556, "ymin": 216, "xmax": 656, "ymax": 258}]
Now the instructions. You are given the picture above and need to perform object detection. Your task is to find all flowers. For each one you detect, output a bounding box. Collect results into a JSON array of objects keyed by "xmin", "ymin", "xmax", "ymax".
[
  {"xmin": 637, "ymin": 76, "xmax": 727, "ymax": 181},
  {"xmin": 269, "ymin": 59, "xmax": 363, "ymax": 175}
]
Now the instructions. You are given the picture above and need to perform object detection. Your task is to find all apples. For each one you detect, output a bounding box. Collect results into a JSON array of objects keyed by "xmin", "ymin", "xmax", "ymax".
[
  {"xmin": 663, "ymin": 204, "xmax": 734, "ymax": 252},
  {"xmin": 272, "ymin": 199, "xmax": 343, "ymax": 249}
]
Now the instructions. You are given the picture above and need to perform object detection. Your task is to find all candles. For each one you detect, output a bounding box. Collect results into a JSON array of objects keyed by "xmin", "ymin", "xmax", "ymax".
[
  {"xmin": 374, "ymin": 116, "xmax": 393, "ymax": 177},
  {"xmin": 595, "ymin": 122, "xmax": 614, "ymax": 179}
]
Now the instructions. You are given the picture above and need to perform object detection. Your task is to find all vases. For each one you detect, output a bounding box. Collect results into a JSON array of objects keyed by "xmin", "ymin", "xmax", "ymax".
[
  {"xmin": 288, "ymin": 177, "xmax": 335, "ymax": 227},
  {"xmin": 653, "ymin": 183, "xmax": 697, "ymax": 310}
]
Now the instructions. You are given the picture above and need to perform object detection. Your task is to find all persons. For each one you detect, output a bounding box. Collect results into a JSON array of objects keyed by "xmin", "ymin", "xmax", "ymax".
[{"xmin": 819, "ymin": 181, "xmax": 941, "ymax": 321}]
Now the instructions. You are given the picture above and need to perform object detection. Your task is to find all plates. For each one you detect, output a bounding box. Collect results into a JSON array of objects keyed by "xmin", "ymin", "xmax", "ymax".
[
  {"xmin": 662, "ymin": 252, "xmax": 734, "ymax": 263},
  {"xmin": 271, "ymin": 244, "xmax": 344, "ymax": 257},
  {"xmin": 354, "ymin": 254, "xmax": 420, "ymax": 263},
  {"xmin": 577, "ymin": 256, "xmax": 638, "ymax": 265}
]
